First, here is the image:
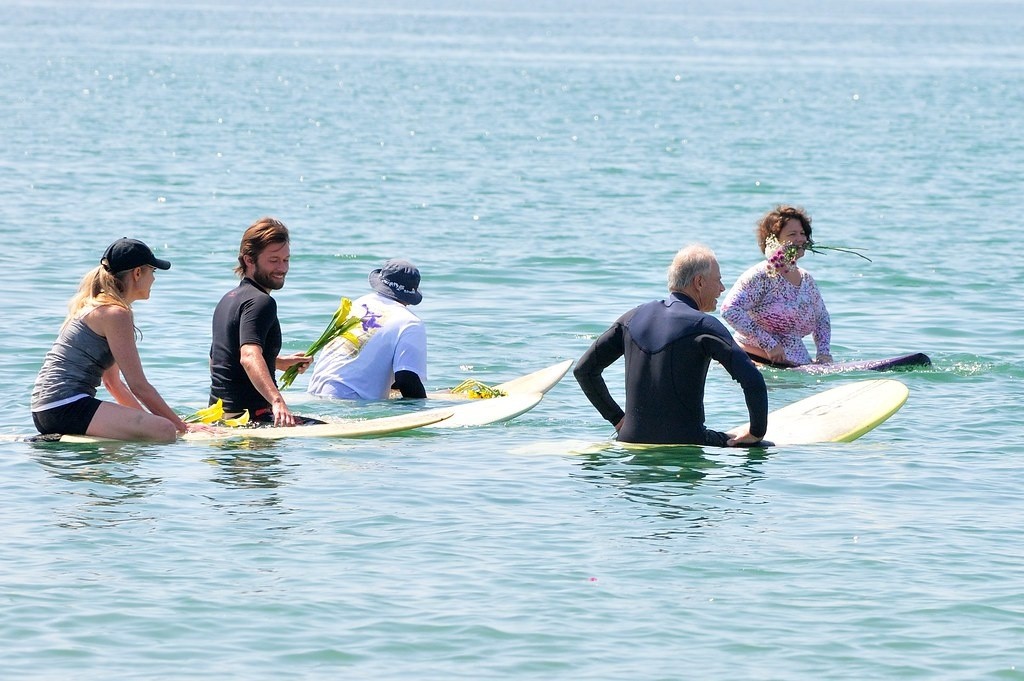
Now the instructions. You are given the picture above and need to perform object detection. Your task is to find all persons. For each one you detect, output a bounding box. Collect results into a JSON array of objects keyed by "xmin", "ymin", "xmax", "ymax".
[
  {"xmin": 571, "ymin": 247, "xmax": 775, "ymax": 448},
  {"xmin": 31, "ymin": 236, "xmax": 186, "ymax": 443},
  {"xmin": 207, "ymin": 219, "xmax": 313, "ymax": 428},
  {"xmin": 307, "ymin": 259, "xmax": 429, "ymax": 400},
  {"xmin": 720, "ymin": 206, "xmax": 834, "ymax": 369}
]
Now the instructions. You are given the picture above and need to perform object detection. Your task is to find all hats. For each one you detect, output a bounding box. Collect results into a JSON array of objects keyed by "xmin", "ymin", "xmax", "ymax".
[
  {"xmin": 368, "ymin": 258, "xmax": 422, "ymax": 305},
  {"xmin": 100, "ymin": 237, "xmax": 171, "ymax": 274}
]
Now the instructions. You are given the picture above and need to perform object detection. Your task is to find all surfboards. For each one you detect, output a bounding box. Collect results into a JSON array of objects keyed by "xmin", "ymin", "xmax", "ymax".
[
  {"xmin": 412, "ymin": 384, "xmax": 540, "ymax": 432},
  {"xmin": 790, "ymin": 352, "xmax": 932, "ymax": 373},
  {"xmin": 429, "ymin": 358, "xmax": 575, "ymax": 398},
  {"xmin": 0, "ymin": 407, "xmax": 453, "ymax": 444},
  {"xmin": 507, "ymin": 378, "xmax": 911, "ymax": 458}
]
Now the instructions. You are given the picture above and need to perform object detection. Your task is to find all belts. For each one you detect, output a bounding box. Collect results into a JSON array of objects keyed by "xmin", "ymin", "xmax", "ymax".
[{"xmin": 255, "ymin": 408, "xmax": 271, "ymax": 416}]
